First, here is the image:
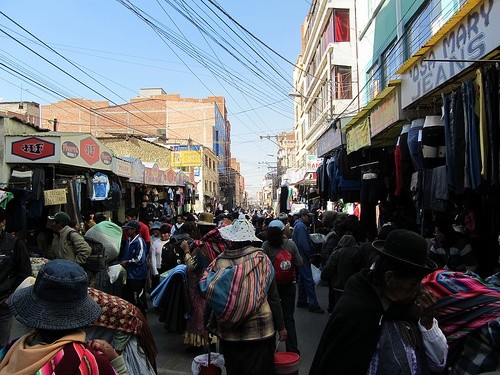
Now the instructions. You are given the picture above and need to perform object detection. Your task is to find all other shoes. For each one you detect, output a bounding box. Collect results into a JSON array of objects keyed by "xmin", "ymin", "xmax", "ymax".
[
  {"xmin": 308, "ymin": 306, "xmax": 324, "ymax": 314},
  {"xmin": 297, "ymin": 301, "xmax": 309, "ymax": 307}
]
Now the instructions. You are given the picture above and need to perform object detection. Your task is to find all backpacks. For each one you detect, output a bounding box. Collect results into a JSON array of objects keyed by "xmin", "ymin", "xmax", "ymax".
[
  {"xmin": 274, "ymin": 239, "xmax": 297, "ymax": 282},
  {"xmin": 67, "ymin": 231, "xmax": 105, "ymax": 272}
]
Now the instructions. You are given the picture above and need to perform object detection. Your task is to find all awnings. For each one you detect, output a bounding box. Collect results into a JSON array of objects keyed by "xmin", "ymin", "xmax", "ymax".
[
  {"xmin": 4, "ymin": 131, "xmax": 189, "ymax": 187},
  {"xmin": 281, "ymin": 0, "xmax": 500, "ymax": 187}
]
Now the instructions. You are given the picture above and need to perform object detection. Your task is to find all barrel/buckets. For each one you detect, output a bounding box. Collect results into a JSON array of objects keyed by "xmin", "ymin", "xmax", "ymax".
[
  {"xmin": 191, "ymin": 336, "xmax": 226, "ymax": 375},
  {"xmin": 273, "ymin": 336, "xmax": 301, "ymax": 375}
]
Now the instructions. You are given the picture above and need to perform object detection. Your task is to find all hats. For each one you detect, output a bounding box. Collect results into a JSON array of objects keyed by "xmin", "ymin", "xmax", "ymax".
[
  {"xmin": 9, "ymin": 259, "xmax": 102, "ymax": 329},
  {"xmin": 122, "ymin": 221, "xmax": 140, "ymax": 231},
  {"xmin": 300, "ymin": 209, "xmax": 315, "ymax": 215},
  {"xmin": 218, "ymin": 219, "xmax": 263, "ymax": 243},
  {"xmin": 268, "ymin": 220, "xmax": 287, "ymax": 230},
  {"xmin": 150, "ymin": 222, "xmax": 162, "ymax": 230},
  {"xmin": 48, "ymin": 212, "xmax": 71, "ymax": 223},
  {"xmin": 372, "ymin": 229, "xmax": 438, "ymax": 274}
]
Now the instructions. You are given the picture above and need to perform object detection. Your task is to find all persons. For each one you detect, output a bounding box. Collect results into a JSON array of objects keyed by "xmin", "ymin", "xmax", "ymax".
[{"xmin": 0, "ymin": 206, "xmax": 500, "ymax": 375}]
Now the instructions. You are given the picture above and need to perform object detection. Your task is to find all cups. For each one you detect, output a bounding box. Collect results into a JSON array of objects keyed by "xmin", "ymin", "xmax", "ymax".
[{"xmin": 409, "ymin": 284, "xmax": 427, "ymax": 303}]
